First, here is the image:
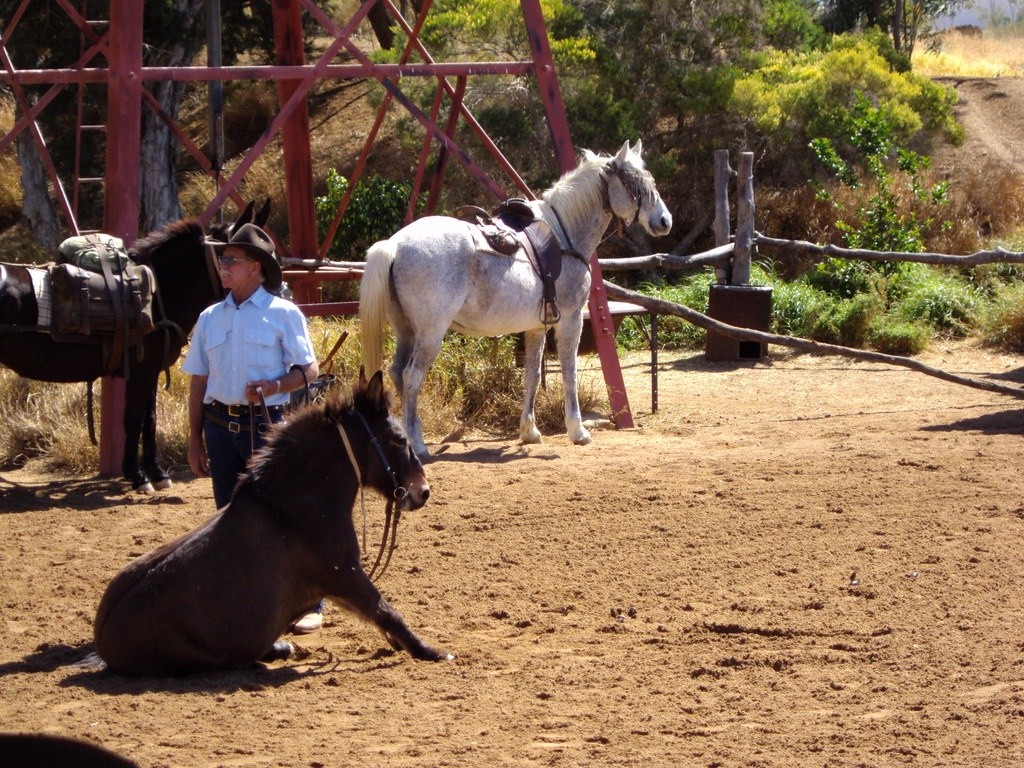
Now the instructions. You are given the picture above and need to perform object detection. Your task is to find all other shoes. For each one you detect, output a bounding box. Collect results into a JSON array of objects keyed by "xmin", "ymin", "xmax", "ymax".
[{"xmin": 295, "ymin": 613, "xmax": 323, "ymax": 633}]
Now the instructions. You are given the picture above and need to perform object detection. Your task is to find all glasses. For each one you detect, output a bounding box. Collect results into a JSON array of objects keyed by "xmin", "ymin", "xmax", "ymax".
[{"xmin": 217, "ymin": 254, "xmax": 253, "ymax": 266}]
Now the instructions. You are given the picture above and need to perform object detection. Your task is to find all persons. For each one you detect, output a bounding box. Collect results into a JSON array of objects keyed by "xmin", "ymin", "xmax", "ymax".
[{"xmin": 184, "ymin": 222, "xmax": 318, "ymax": 513}]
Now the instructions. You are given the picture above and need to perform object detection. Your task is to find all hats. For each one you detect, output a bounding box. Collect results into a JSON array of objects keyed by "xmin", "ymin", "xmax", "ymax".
[{"xmin": 204, "ymin": 223, "xmax": 283, "ymax": 291}]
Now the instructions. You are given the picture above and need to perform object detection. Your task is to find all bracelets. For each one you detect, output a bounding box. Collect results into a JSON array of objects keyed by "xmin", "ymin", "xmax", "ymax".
[{"xmin": 274, "ymin": 378, "xmax": 283, "ymax": 394}]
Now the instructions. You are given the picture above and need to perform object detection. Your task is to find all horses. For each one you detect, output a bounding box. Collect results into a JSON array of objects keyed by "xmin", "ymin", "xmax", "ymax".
[
  {"xmin": 358, "ymin": 138, "xmax": 673, "ymax": 461},
  {"xmin": 72, "ymin": 364, "xmax": 454, "ymax": 673},
  {"xmin": 0, "ymin": 198, "xmax": 273, "ymax": 492}
]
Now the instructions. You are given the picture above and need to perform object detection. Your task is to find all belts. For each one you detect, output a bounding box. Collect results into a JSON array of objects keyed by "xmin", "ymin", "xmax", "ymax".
[
  {"xmin": 203, "ymin": 410, "xmax": 259, "ymax": 433},
  {"xmin": 205, "ymin": 400, "xmax": 282, "ymax": 417}
]
